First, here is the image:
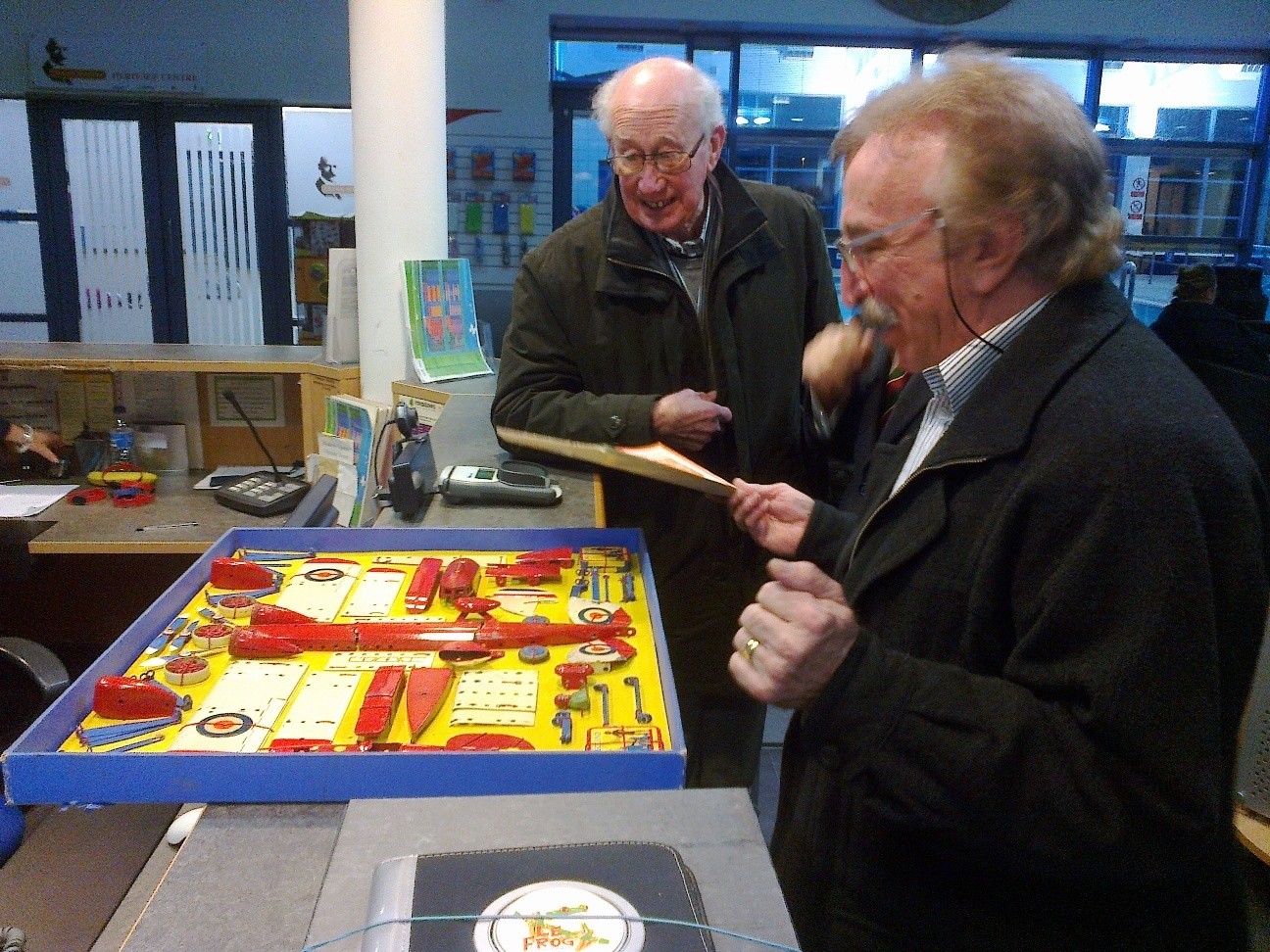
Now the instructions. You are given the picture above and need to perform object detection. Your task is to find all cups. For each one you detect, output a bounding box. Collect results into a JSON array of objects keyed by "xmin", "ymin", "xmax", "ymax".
[{"xmin": 72, "ymin": 436, "xmax": 111, "ymax": 473}]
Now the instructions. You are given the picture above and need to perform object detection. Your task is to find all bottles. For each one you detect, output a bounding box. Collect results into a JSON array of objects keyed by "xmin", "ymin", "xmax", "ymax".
[{"xmin": 108, "ymin": 405, "xmax": 134, "ymax": 472}]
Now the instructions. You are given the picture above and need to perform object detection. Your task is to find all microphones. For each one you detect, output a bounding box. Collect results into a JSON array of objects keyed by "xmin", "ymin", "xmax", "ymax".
[{"xmin": 221, "ymin": 388, "xmax": 282, "ymax": 482}]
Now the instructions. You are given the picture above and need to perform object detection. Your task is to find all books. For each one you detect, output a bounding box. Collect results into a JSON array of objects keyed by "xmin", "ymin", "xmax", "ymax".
[{"xmin": 305, "ymin": 392, "xmax": 394, "ymax": 530}]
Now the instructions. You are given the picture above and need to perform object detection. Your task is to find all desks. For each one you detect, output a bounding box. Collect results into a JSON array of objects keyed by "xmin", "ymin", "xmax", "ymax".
[{"xmin": 0, "ymin": 469, "xmax": 300, "ymax": 556}]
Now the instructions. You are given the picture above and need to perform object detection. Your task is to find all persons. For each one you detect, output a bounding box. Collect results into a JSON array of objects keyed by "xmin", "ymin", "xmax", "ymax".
[
  {"xmin": 0, "ymin": 414, "xmax": 63, "ymax": 465},
  {"xmin": 42, "ymin": 36, "xmax": 75, "ymax": 86},
  {"xmin": 491, "ymin": 57, "xmax": 845, "ymax": 790},
  {"xmin": 781, "ymin": 299, "xmax": 937, "ymax": 510},
  {"xmin": 1147, "ymin": 261, "xmax": 1246, "ymax": 379},
  {"xmin": 315, "ymin": 155, "xmax": 343, "ymax": 200},
  {"xmin": 726, "ymin": 49, "xmax": 1270, "ymax": 952}
]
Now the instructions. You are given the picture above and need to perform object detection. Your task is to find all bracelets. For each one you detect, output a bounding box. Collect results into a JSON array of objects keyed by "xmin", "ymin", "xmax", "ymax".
[{"xmin": 11, "ymin": 422, "xmax": 34, "ymax": 454}]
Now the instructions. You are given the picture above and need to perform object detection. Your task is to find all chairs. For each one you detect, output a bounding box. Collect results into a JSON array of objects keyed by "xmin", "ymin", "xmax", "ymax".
[{"xmin": 1, "ymin": 634, "xmax": 72, "ymax": 868}]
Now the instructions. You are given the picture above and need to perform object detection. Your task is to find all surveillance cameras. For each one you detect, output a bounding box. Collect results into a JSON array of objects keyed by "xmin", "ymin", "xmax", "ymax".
[{"xmin": 393, "ymin": 400, "xmax": 420, "ymax": 439}]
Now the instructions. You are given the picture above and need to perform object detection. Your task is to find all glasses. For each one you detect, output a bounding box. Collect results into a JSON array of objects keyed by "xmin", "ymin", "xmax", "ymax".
[
  {"xmin": 836, "ymin": 208, "xmax": 938, "ymax": 274},
  {"xmin": 606, "ymin": 133, "xmax": 705, "ymax": 175}
]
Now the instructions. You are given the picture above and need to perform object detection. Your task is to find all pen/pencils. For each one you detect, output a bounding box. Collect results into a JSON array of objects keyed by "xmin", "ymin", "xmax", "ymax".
[{"xmin": 135, "ymin": 520, "xmax": 200, "ymax": 531}]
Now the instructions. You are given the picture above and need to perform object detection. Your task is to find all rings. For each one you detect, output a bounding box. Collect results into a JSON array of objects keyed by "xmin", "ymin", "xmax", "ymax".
[{"xmin": 743, "ymin": 638, "xmax": 761, "ymax": 661}]
